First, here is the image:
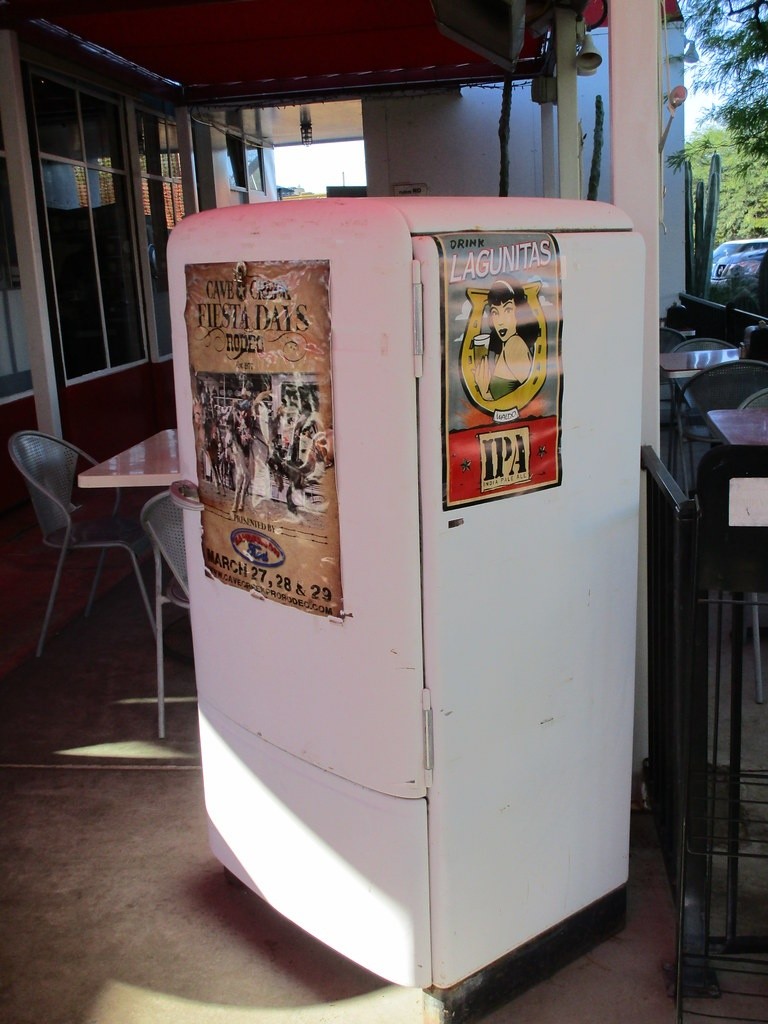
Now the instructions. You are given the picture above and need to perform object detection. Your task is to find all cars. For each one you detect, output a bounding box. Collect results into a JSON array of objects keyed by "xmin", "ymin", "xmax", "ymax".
[{"xmin": 710, "ymin": 248, "xmax": 768, "ymax": 285}]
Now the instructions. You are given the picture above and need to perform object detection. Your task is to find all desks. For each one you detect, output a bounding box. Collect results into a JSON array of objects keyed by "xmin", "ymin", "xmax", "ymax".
[
  {"xmin": 660, "ymin": 349, "xmax": 739, "ymax": 372},
  {"xmin": 704, "ymin": 409, "xmax": 768, "ymax": 445},
  {"xmin": 728, "ymin": 476, "xmax": 768, "ymax": 527},
  {"xmin": 78, "ymin": 429, "xmax": 181, "ymax": 489}
]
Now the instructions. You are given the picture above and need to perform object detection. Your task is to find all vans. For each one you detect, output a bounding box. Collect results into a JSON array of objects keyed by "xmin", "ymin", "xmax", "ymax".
[{"xmin": 712, "ymin": 238, "xmax": 768, "ymax": 265}]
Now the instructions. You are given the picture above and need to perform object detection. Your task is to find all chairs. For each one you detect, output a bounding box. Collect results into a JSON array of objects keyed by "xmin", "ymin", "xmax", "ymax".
[
  {"xmin": 7, "ymin": 430, "xmax": 157, "ymax": 657},
  {"xmin": 670, "ymin": 358, "xmax": 768, "ymax": 499},
  {"xmin": 670, "ymin": 338, "xmax": 738, "ymax": 490},
  {"xmin": 660, "ymin": 328, "xmax": 686, "ymax": 427},
  {"xmin": 138, "ymin": 492, "xmax": 191, "ymax": 740}
]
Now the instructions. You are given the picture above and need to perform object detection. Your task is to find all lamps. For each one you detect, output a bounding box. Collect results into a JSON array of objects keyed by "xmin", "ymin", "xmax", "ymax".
[
  {"xmin": 300, "ymin": 123, "xmax": 313, "ymax": 145},
  {"xmin": 576, "ymin": 34, "xmax": 602, "ymax": 76}
]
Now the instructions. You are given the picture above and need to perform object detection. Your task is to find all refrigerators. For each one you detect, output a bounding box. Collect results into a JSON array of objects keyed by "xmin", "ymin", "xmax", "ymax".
[{"xmin": 167, "ymin": 196, "xmax": 645, "ymax": 988}]
